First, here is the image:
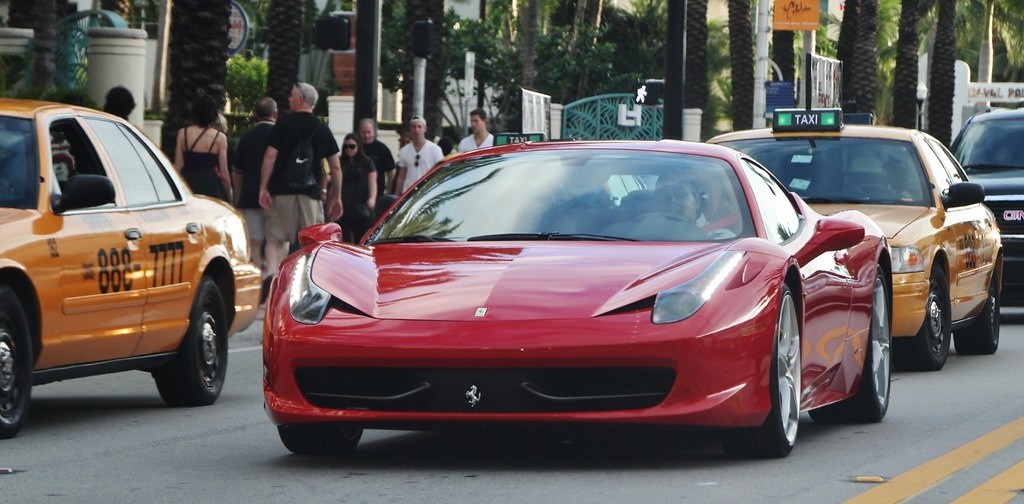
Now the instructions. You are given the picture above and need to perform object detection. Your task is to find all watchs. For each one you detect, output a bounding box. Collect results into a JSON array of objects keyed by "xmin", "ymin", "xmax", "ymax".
[{"xmin": 321, "ymin": 187, "xmax": 330, "ymax": 195}]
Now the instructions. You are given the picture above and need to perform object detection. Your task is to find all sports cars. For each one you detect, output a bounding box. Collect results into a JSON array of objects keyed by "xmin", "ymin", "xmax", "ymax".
[
  {"xmin": 705, "ymin": 105, "xmax": 1007, "ymax": 370},
  {"xmin": 267, "ymin": 139, "xmax": 897, "ymax": 466}
]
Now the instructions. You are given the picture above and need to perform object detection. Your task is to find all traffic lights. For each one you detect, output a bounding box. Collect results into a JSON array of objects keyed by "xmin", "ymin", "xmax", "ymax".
[{"xmin": 634, "ymin": 79, "xmax": 660, "ymax": 105}]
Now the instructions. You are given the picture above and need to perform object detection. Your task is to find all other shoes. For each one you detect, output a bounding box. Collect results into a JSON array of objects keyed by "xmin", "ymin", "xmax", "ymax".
[{"xmin": 261, "ymin": 274, "xmax": 272, "ymax": 305}]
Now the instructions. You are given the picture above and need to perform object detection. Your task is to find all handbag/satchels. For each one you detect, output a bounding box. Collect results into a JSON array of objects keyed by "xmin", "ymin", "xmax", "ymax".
[{"xmin": 282, "ymin": 141, "xmax": 314, "ymax": 184}]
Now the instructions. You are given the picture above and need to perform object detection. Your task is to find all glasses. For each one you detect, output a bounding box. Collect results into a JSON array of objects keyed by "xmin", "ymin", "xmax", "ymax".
[
  {"xmin": 344, "ymin": 144, "xmax": 356, "ymax": 149},
  {"xmin": 415, "ymin": 155, "xmax": 420, "ymax": 167},
  {"xmin": 294, "ymin": 80, "xmax": 305, "ymax": 97},
  {"xmin": 657, "ymin": 188, "xmax": 690, "ymax": 203}
]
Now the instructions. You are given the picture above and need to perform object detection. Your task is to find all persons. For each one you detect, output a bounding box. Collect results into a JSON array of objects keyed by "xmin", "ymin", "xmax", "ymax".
[
  {"xmin": 389, "ymin": 114, "xmax": 458, "ymax": 197},
  {"xmin": 629, "ymin": 172, "xmax": 737, "ymax": 241},
  {"xmin": 231, "ymin": 97, "xmax": 280, "ymax": 280},
  {"xmin": 456, "ymin": 109, "xmax": 494, "ymax": 154},
  {"xmin": 160, "ymin": 71, "xmax": 234, "ymax": 214},
  {"xmin": 356, "ymin": 117, "xmax": 396, "ymax": 199},
  {"xmin": 258, "ymin": 83, "xmax": 344, "ymax": 307},
  {"xmin": 100, "ymin": 85, "xmax": 136, "ymax": 124},
  {"xmin": 320, "ymin": 132, "xmax": 378, "ymax": 246}
]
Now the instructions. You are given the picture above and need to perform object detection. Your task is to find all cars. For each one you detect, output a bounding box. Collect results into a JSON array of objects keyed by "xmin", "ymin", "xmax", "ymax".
[
  {"xmin": 0, "ymin": 97, "xmax": 263, "ymax": 436},
  {"xmin": 946, "ymin": 82, "xmax": 1024, "ymax": 318}
]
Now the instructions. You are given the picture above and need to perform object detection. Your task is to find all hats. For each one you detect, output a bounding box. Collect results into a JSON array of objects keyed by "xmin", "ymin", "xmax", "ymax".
[{"xmin": 410, "ymin": 115, "xmax": 426, "ymax": 125}]
